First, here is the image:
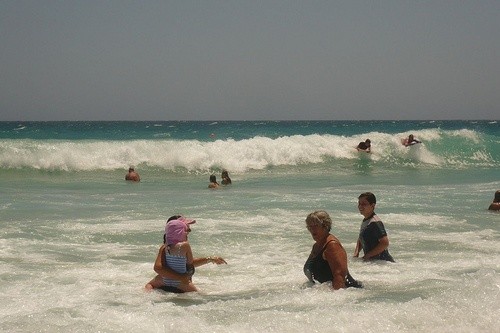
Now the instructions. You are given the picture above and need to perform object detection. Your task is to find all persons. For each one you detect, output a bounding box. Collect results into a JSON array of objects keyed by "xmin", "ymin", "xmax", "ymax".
[
  {"xmin": 401, "ymin": 134, "xmax": 422, "ymax": 147},
  {"xmin": 487, "ymin": 190, "xmax": 500, "ymax": 212},
  {"xmin": 356, "ymin": 139, "xmax": 371, "ymax": 153},
  {"xmin": 208, "ymin": 174, "xmax": 220, "ymax": 189},
  {"xmin": 352, "ymin": 192, "xmax": 396, "ymax": 263},
  {"xmin": 146, "ymin": 215, "xmax": 228, "ymax": 294},
  {"xmin": 303, "ymin": 209, "xmax": 363, "ymax": 290},
  {"xmin": 125, "ymin": 167, "xmax": 140, "ymax": 182},
  {"xmin": 221, "ymin": 170, "xmax": 231, "ymax": 184}
]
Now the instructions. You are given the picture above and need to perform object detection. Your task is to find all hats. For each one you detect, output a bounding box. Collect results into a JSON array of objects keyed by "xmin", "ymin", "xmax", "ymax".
[
  {"xmin": 176, "ymin": 216, "xmax": 195, "ymax": 224},
  {"xmin": 165, "ymin": 220, "xmax": 188, "ymax": 245}
]
